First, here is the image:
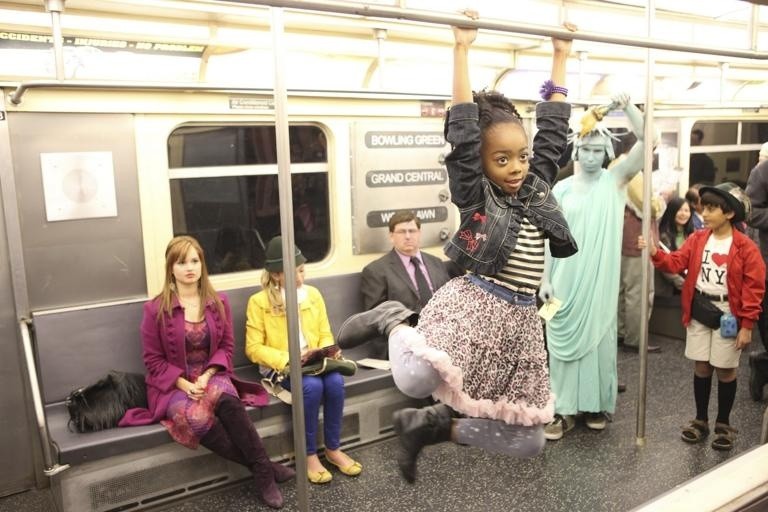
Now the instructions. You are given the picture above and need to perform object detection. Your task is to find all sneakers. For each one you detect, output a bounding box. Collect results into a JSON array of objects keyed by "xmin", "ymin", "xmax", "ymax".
[
  {"xmin": 323, "ymin": 446, "xmax": 363, "ymax": 477},
  {"xmin": 582, "ymin": 411, "xmax": 608, "ymax": 431},
  {"xmin": 543, "ymin": 412, "xmax": 577, "ymax": 441},
  {"xmin": 748, "ymin": 349, "xmax": 768, "ymax": 402},
  {"xmin": 307, "ymin": 467, "xmax": 333, "ymax": 485}
]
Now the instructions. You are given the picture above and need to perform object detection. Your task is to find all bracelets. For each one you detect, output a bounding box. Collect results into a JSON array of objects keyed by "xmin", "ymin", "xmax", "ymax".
[{"xmin": 539, "ymin": 80, "xmax": 568, "ymax": 101}]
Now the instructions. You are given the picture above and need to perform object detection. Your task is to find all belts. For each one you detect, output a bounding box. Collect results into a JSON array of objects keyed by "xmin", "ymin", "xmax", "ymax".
[{"xmin": 694, "ymin": 287, "xmax": 729, "ymax": 302}]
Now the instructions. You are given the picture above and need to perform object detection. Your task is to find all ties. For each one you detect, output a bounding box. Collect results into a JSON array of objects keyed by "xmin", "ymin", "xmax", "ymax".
[{"xmin": 409, "ymin": 255, "xmax": 434, "ymax": 308}]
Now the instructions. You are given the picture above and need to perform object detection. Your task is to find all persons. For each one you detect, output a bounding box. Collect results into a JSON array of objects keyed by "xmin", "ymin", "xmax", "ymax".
[
  {"xmin": 360, "ymin": 210, "xmax": 451, "ymax": 409},
  {"xmin": 538, "ymin": 91, "xmax": 661, "ymax": 442},
  {"xmin": 117, "ymin": 235, "xmax": 296, "ymax": 509},
  {"xmin": 336, "ymin": 10, "xmax": 579, "ymax": 485},
  {"xmin": 244, "ymin": 236, "xmax": 363, "ymax": 484}
]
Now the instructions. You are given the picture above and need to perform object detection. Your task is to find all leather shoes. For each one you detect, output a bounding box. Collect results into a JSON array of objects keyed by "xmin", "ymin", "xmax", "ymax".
[{"xmin": 617, "ymin": 336, "xmax": 663, "ymax": 354}]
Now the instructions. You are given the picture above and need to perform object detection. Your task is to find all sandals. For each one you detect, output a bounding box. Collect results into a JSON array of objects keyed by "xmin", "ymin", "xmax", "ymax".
[
  {"xmin": 679, "ymin": 419, "xmax": 711, "ymax": 444},
  {"xmin": 710, "ymin": 422, "xmax": 739, "ymax": 452}
]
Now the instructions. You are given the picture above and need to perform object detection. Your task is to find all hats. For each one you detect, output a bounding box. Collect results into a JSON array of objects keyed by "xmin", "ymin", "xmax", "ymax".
[
  {"xmin": 698, "ymin": 181, "xmax": 753, "ymax": 224},
  {"xmin": 261, "ymin": 235, "xmax": 308, "ymax": 274}
]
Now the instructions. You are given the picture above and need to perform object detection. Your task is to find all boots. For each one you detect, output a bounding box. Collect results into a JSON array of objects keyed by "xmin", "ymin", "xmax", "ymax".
[
  {"xmin": 214, "ymin": 396, "xmax": 285, "ymax": 509},
  {"xmin": 390, "ymin": 402, "xmax": 454, "ymax": 486},
  {"xmin": 337, "ymin": 299, "xmax": 420, "ymax": 351},
  {"xmin": 197, "ymin": 417, "xmax": 297, "ymax": 485}
]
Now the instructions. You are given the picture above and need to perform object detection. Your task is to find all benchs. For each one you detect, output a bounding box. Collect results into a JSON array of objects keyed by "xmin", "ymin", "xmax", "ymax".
[{"xmin": 17, "ymin": 260, "xmax": 463, "ymax": 512}]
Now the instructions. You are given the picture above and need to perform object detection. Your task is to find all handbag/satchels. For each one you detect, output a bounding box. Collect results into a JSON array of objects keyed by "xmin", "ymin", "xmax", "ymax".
[
  {"xmin": 259, "ymin": 352, "xmax": 359, "ymax": 407},
  {"xmin": 64, "ymin": 369, "xmax": 149, "ymax": 435},
  {"xmin": 690, "ymin": 291, "xmax": 725, "ymax": 331}
]
